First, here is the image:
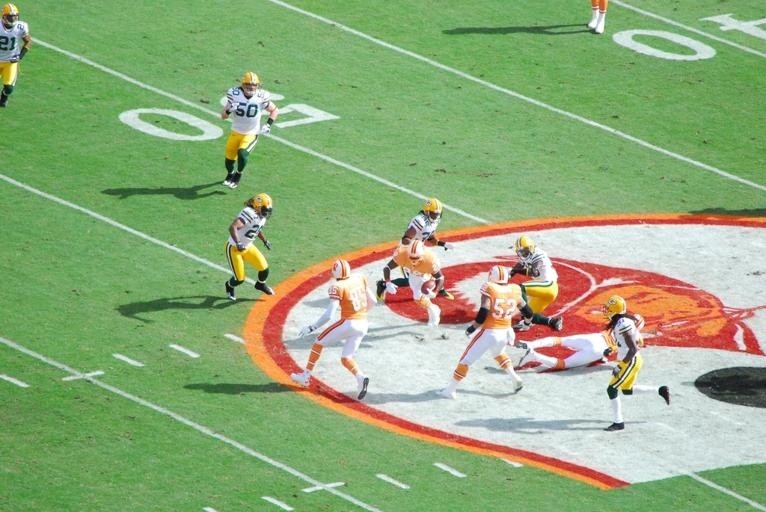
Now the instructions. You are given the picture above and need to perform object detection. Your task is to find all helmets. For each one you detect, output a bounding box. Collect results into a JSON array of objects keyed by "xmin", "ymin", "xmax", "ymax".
[
  {"xmin": 2, "ymin": 2, "xmax": 18, "ymax": 27},
  {"xmin": 632, "ymin": 314, "xmax": 644, "ymax": 330},
  {"xmin": 603, "ymin": 296, "xmax": 626, "ymax": 320},
  {"xmin": 514, "ymin": 237, "xmax": 535, "ymax": 265},
  {"xmin": 253, "ymin": 192, "xmax": 273, "ymax": 219},
  {"xmin": 332, "ymin": 259, "xmax": 350, "ymax": 281},
  {"xmin": 408, "ymin": 240, "xmax": 423, "ymax": 266},
  {"xmin": 489, "ymin": 265, "xmax": 509, "ymax": 285},
  {"xmin": 241, "ymin": 72, "xmax": 260, "ymax": 98},
  {"xmin": 424, "ymin": 197, "xmax": 442, "ymax": 224}
]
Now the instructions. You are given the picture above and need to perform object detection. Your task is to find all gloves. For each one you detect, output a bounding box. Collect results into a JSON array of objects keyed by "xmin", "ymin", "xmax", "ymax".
[
  {"xmin": 385, "ymin": 280, "xmax": 398, "ymax": 296},
  {"xmin": 260, "ymin": 117, "xmax": 274, "ymax": 134},
  {"xmin": 519, "ymin": 323, "xmax": 530, "ymax": 332},
  {"xmin": 611, "ymin": 365, "xmax": 622, "ymax": 378},
  {"xmin": 465, "ymin": 325, "xmax": 479, "ymax": 336},
  {"xmin": 423, "ymin": 289, "xmax": 436, "ymax": 300},
  {"xmin": 298, "ymin": 324, "xmax": 315, "ymax": 338},
  {"xmin": 604, "ymin": 349, "xmax": 611, "ymax": 356},
  {"xmin": 226, "ymin": 100, "xmax": 239, "ymax": 115},
  {"xmin": 237, "ymin": 242, "xmax": 245, "ymax": 251},
  {"xmin": 264, "ymin": 240, "xmax": 271, "ymax": 250},
  {"xmin": 10, "ymin": 54, "xmax": 20, "ymax": 63},
  {"xmin": 437, "ymin": 239, "xmax": 455, "ymax": 251}
]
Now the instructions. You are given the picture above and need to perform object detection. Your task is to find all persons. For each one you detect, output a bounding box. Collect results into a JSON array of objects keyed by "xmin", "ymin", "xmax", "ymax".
[
  {"xmin": 588, "ymin": 0, "xmax": 609, "ymax": 34},
  {"xmin": 224, "ymin": 191, "xmax": 277, "ymax": 301},
  {"xmin": 220, "ymin": 71, "xmax": 280, "ymax": 189},
  {"xmin": 0, "ymin": 2, "xmax": 33, "ymax": 107}
]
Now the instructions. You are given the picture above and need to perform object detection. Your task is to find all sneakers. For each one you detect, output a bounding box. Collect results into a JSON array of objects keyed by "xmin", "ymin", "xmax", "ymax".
[
  {"xmin": 230, "ymin": 172, "xmax": 242, "ymax": 189},
  {"xmin": 427, "ymin": 305, "xmax": 442, "ymax": 329},
  {"xmin": 515, "ymin": 339, "xmax": 532, "ymax": 393},
  {"xmin": 605, "ymin": 421, "xmax": 624, "ymax": 431},
  {"xmin": 223, "ymin": 172, "xmax": 236, "ymax": 186},
  {"xmin": 548, "ymin": 317, "xmax": 563, "ymax": 331},
  {"xmin": 289, "ymin": 372, "xmax": 310, "ymax": 386},
  {"xmin": 357, "ymin": 376, "xmax": 369, "ymax": 400},
  {"xmin": 225, "ymin": 280, "xmax": 236, "ymax": 300},
  {"xmin": 377, "ymin": 279, "xmax": 385, "ymax": 303},
  {"xmin": 588, "ymin": 19, "xmax": 604, "ymax": 33},
  {"xmin": 255, "ymin": 281, "xmax": 273, "ymax": 294},
  {"xmin": 659, "ymin": 386, "xmax": 670, "ymax": 405},
  {"xmin": 0, "ymin": 90, "xmax": 8, "ymax": 108},
  {"xmin": 435, "ymin": 387, "xmax": 457, "ymax": 399},
  {"xmin": 439, "ymin": 288, "xmax": 454, "ymax": 300}
]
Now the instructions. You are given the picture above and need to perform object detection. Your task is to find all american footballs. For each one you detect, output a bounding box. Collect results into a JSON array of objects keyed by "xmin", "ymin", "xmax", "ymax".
[{"xmin": 422, "ymin": 280, "xmax": 437, "ymax": 294}]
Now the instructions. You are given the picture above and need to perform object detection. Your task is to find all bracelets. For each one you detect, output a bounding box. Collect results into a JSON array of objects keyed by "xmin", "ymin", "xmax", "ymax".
[
  {"xmin": 225, "ymin": 109, "xmax": 232, "ymax": 115},
  {"xmin": 267, "ymin": 118, "xmax": 273, "ymax": 127}
]
